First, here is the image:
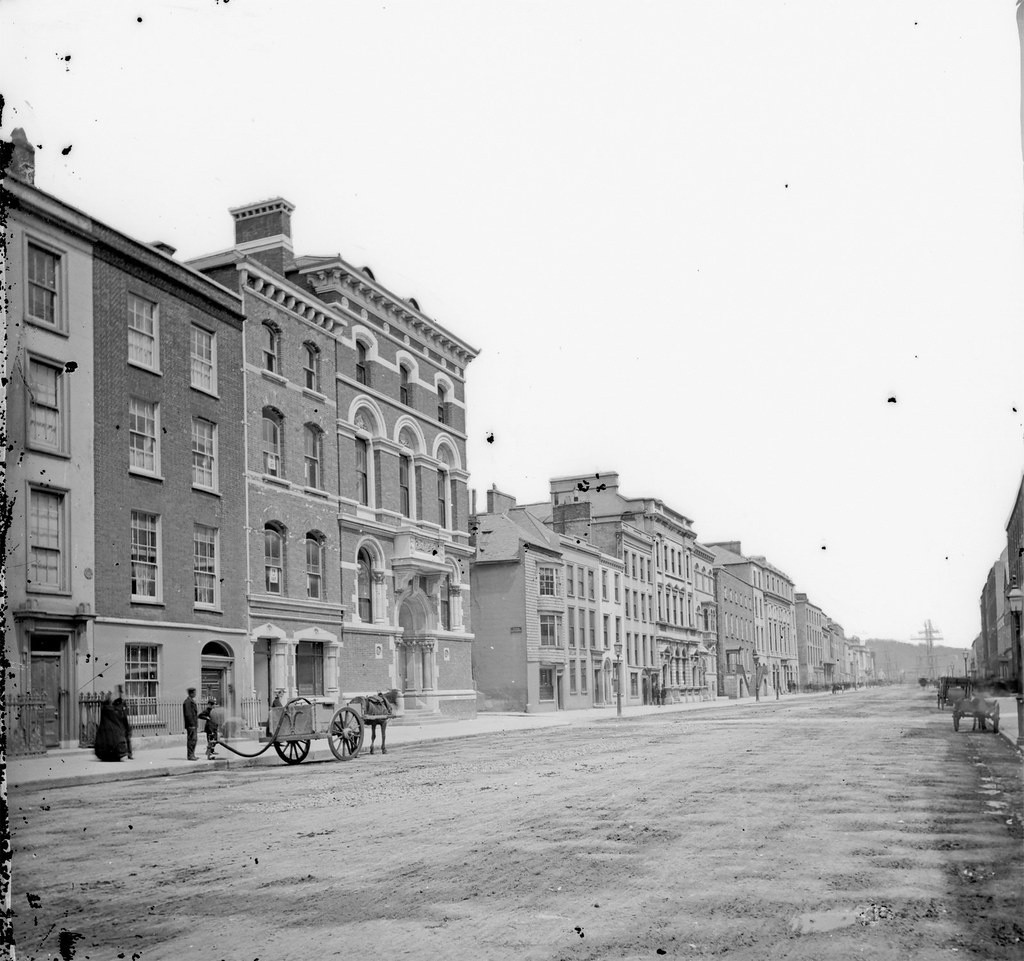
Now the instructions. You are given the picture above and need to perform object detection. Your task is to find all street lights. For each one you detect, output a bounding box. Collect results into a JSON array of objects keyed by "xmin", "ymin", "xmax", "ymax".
[
  {"xmin": 614, "ymin": 639, "xmax": 623, "ymax": 716},
  {"xmin": 776, "ymin": 664, "xmax": 780, "ymax": 700},
  {"xmin": 962, "ymin": 647, "xmax": 969, "ymax": 677},
  {"xmin": 1006, "ymin": 573, "xmax": 1024, "ymax": 747},
  {"xmin": 950, "ymin": 661, "xmax": 954, "ymax": 677},
  {"xmin": 753, "ymin": 653, "xmax": 760, "ymax": 702}
]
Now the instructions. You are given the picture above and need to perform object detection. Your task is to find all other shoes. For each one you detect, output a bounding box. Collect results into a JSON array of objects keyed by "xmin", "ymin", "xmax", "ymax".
[
  {"xmin": 191, "ymin": 756, "xmax": 200, "ymax": 760},
  {"xmin": 208, "ymin": 754, "xmax": 216, "ymax": 759}
]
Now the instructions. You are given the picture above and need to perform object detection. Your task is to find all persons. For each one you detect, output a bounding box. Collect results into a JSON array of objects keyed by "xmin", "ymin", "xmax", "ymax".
[
  {"xmin": 198, "ymin": 699, "xmax": 218, "ymax": 755},
  {"xmin": 652, "ymin": 683, "xmax": 666, "ymax": 705},
  {"xmin": 183, "ymin": 688, "xmax": 199, "ymax": 760},
  {"xmin": 94, "ymin": 685, "xmax": 133, "ymax": 762}
]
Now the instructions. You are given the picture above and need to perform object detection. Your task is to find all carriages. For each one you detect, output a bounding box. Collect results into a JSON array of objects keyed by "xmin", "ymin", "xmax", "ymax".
[
  {"xmin": 258, "ymin": 687, "xmax": 400, "ymax": 763},
  {"xmin": 936, "ymin": 677, "xmax": 973, "ymax": 710},
  {"xmin": 952, "ymin": 691, "xmax": 1001, "ymax": 734}
]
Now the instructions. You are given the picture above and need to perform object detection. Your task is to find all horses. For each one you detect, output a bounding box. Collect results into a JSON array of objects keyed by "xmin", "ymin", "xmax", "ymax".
[{"xmin": 346, "ymin": 689, "xmax": 401, "ymax": 758}]
